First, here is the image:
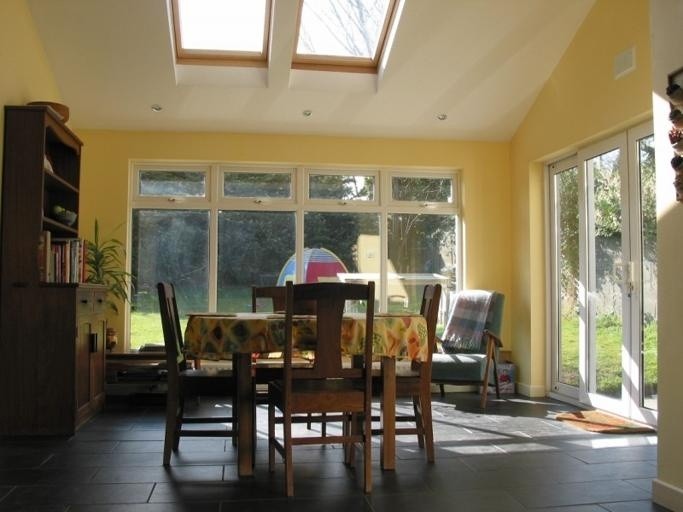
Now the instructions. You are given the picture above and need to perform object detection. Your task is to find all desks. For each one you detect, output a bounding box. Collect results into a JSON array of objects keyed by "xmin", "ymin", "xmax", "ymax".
[{"xmin": 184, "ymin": 312, "xmax": 428, "ymax": 478}]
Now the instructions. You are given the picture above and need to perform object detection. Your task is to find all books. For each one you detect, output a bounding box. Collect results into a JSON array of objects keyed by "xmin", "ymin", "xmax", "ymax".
[{"xmin": 38, "ymin": 230, "xmax": 88, "ymax": 283}]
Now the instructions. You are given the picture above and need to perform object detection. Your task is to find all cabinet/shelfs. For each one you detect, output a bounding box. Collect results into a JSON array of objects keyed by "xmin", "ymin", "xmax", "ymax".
[{"xmin": 0, "ymin": 105, "xmax": 110, "ymax": 449}]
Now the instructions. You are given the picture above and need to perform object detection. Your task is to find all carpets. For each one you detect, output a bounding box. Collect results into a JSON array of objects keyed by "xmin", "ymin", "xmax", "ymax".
[{"xmin": 553, "ymin": 410, "xmax": 654, "ymax": 433}]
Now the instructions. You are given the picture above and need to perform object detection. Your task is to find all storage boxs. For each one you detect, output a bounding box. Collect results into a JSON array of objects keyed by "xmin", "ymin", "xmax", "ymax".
[{"xmin": 478, "ymin": 350, "xmax": 516, "ymax": 394}]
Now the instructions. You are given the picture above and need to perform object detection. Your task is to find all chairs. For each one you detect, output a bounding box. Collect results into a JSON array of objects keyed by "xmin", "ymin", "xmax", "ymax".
[
  {"xmin": 430, "ymin": 288, "xmax": 503, "ymax": 404},
  {"xmin": 269, "ymin": 281, "xmax": 377, "ymax": 497},
  {"xmin": 251, "ymin": 287, "xmax": 328, "ymax": 441},
  {"xmin": 344, "ymin": 283, "xmax": 442, "ymax": 467},
  {"xmin": 155, "ymin": 280, "xmax": 239, "ymax": 467}
]
